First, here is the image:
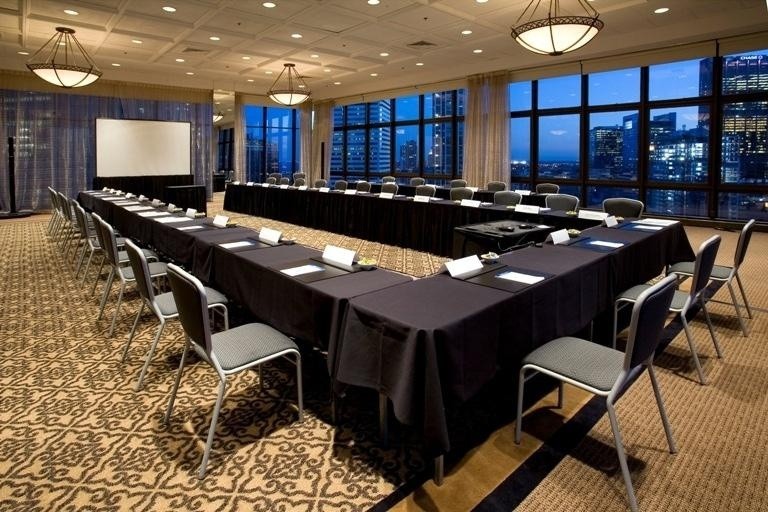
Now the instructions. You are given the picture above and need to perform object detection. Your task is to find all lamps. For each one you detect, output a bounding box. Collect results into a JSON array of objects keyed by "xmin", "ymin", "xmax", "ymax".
[
  {"xmin": 22, "ymin": 25, "xmax": 103, "ymax": 89},
  {"xmin": 508, "ymin": 0, "xmax": 605, "ymax": 56},
  {"xmin": 266, "ymin": 62, "xmax": 314, "ymax": 108}
]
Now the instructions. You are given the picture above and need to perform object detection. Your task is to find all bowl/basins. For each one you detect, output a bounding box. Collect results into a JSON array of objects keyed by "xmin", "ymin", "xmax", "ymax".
[
  {"xmin": 226, "ymin": 222, "xmax": 236, "ymax": 228},
  {"xmin": 406, "ymin": 196, "xmax": 414, "ymax": 201},
  {"xmin": 281, "ymin": 234, "xmax": 297, "ymax": 246},
  {"xmin": 507, "ymin": 205, "xmax": 518, "ymax": 211},
  {"xmin": 455, "ymin": 199, "xmax": 461, "ymax": 205},
  {"xmin": 373, "ymin": 192, "xmax": 380, "ymax": 198},
  {"xmin": 159, "ymin": 202, "xmax": 166, "ymax": 207},
  {"xmin": 172, "ymin": 207, "xmax": 184, "ymax": 212},
  {"xmin": 143, "ymin": 197, "xmax": 149, "ymax": 202},
  {"xmin": 616, "ymin": 215, "xmax": 624, "ymax": 225},
  {"xmin": 358, "ymin": 258, "xmax": 376, "ymax": 271},
  {"xmin": 481, "ymin": 251, "xmax": 501, "ymax": 264},
  {"xmin": 195, "ymin": 211, "xmax": 206, "ymax": 219},
  {"xmin": 568, "ymin": 229, "xmax": 581, "ymax": 237},
  {"xmin": 566, "ymin": 210, "xmax": 578, "ymax": 217}
]
